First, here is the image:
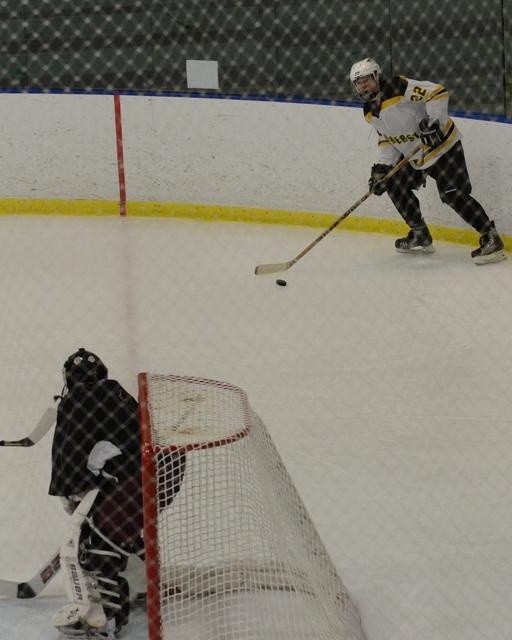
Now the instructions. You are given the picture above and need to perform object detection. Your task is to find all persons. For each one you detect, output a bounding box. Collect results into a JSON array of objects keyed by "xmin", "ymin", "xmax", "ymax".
[
  {"xmin": 351, "ymin": 57, "xmax": 504, "ymax": 258},
  {"xmin": 48, "ymin": 348, "xmax": 185, "ymax": 637}
]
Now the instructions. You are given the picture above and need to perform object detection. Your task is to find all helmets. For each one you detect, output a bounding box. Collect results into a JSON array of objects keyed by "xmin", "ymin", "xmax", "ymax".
[
  {"xmin": 61, "ymin": 348, "xmax": 106, "ymax": 391},
  {"xmin": 350, "ymin": 58, "xmax": 383, "ymax": 104}
]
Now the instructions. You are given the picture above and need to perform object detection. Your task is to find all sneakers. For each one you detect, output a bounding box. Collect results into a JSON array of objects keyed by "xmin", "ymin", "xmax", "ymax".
[{"xmin": 58, "ymin": 616, "xmax": 117, "ymax": 636}]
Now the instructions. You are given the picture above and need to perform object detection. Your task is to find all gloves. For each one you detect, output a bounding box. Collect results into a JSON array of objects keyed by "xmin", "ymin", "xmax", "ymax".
[
  {"xmin": 419, "ymin": 118, "xmax": 445, "ymax": 147},
  {"xmin": 368, "ymin": 163, "xmax": 396, "ymax": 196}
]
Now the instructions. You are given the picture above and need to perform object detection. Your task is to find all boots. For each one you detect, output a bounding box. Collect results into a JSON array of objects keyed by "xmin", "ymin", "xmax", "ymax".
[
  {"xmin": 471, "ymin": 221, "xmax": 504, "ymax": 257},
  {"xmin": 396, "ymin": 226, "xmax": 432, "ymax": 248}
]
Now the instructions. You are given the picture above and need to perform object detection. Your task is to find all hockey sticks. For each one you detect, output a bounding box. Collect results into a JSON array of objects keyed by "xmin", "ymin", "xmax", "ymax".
[
  {"xmin": 0, "ymin": 395, "xmax": 203, "ymax": 599},
  {"xmin": 0, "ymin": 405, "xmax": 203, "ymax": 599},
  {"xmin": 255, "ymin": 141, "xmax": 422, "ymax": 274}
]
